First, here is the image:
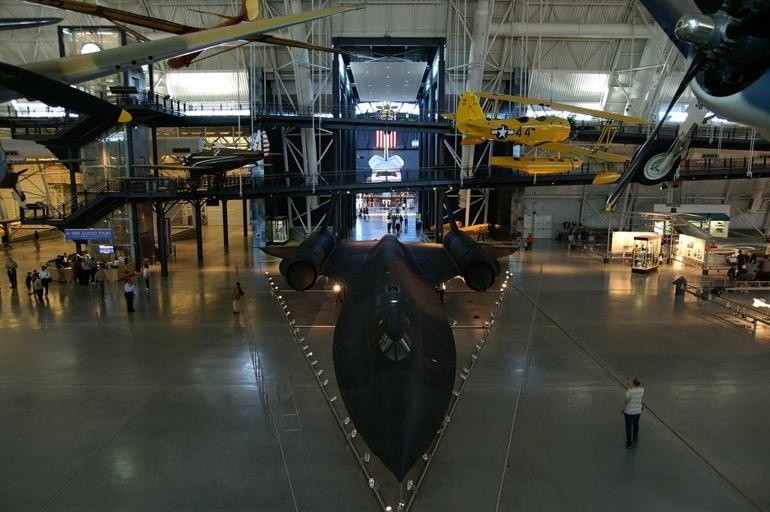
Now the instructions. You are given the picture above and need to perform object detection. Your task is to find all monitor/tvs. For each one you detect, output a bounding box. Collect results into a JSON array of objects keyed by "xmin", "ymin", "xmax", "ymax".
[{"xmin": 98, "ymin": 244, "xmax": 115, "ymax": 254}]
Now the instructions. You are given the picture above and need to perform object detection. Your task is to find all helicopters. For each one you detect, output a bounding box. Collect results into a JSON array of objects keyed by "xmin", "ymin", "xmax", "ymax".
[{"xmin": 441, "ymin": 90, "xmax": 650, "ymax": 185}]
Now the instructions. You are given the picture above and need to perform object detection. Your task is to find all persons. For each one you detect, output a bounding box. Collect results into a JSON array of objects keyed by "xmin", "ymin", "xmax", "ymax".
[
  {"xmin": 25, "ymin": 252, "xmax": 106, "ymax": 300},
  {"xmin": 33, "ymin": 230, "xmax": 41, "ymax": 252},
  {"xmin": 5, "ymin": 256, "xmax": 18, "ymax": 289},
  {"xmin": 140, "ymin": 257, "xmax": 151, "ymax": 290},
  {"xmin": 726, "ymin": 263, "xmax": 736, "ymax": 280},
  {"xmin": 231, "ymin": 281, "xmax": 245, "ymax": 315},
  {"xmin": 524, "ymin": 231, "xmax": 534, "ymax": 252},
  {"xmin": 737, "ymin": 249, "xmax": 745, "ymax": 267},
  {"xmin": 122, "ymin": 278, "xmax": 136, "ymax": 312},
  {"xmin": 359, "ymin": 204, "xmax": 408, "ymax": 235},
  {"xmin": 620, "ymin": 375, "xmax": 644, "ymax": 448}
]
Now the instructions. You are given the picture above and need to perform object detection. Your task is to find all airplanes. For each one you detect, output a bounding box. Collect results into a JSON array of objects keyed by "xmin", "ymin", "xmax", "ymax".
[{"xmin": 602, "ymin": 0, "xmax": 770, "ymax": 214}]
